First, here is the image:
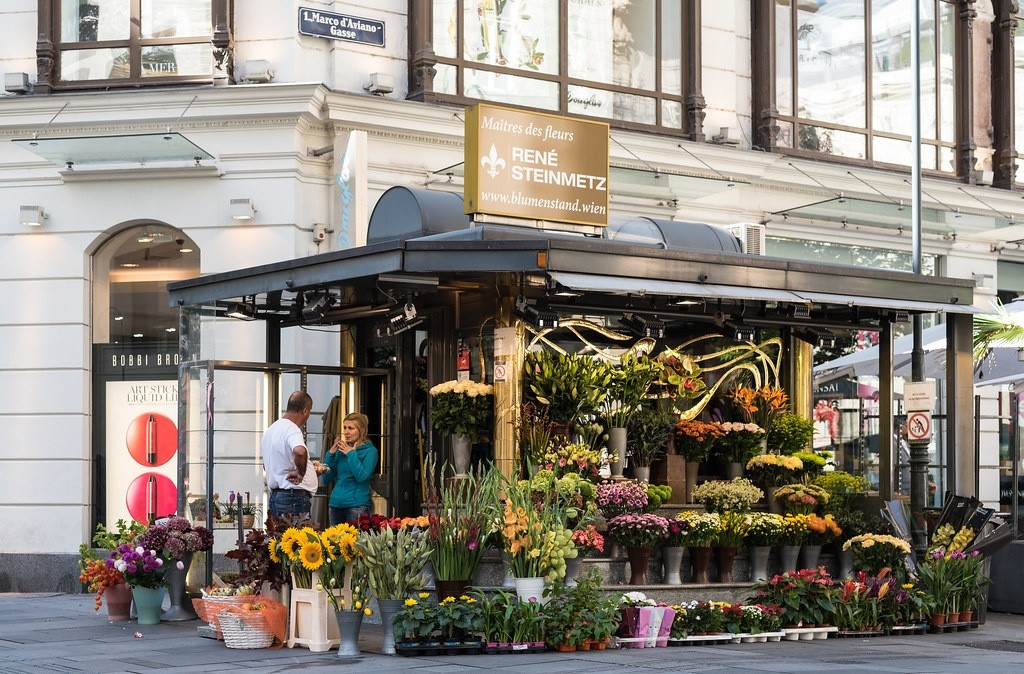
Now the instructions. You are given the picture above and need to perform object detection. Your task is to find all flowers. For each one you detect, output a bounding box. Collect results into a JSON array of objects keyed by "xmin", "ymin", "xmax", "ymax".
[
  {"xmin": 269, "ymin": 351, "xmax": 987, "ymax": 605},
  {"xmin": 77, "ymin": 514, "xmax": 214, "ymax": 612}
]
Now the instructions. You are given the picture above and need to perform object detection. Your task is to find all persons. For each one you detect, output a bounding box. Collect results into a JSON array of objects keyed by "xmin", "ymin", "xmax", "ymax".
[
  {"xmin": 322, "ymin": 412, "xmax": 378, "ymax": 538},
  {"xmin": 261, "ymin": 391, "xmax": 319, "ymax": 538}
]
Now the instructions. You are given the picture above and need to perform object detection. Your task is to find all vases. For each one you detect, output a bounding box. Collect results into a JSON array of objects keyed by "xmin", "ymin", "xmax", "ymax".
[
  {"xmin": 713, "ymin": 547, "xmax": 737, "ymax": 582},
  {"xmin": 804, "ymin": 546, "xmax": 822, "ymax": 568},
  {"xmin": 627, "ymin": 548, "xmax": 652, "ymax": 585},
  {"xmin": 608, "ymin": 427, "xmax": 628, "ymax": 478},
  {"xmin": 104, "ymin": 551, "xmax": 196, "ymax": 625},
  {"xmin": 656, "ymin": 454, "xmax": 687, "ymax": 504},
  {"xmin": 688, "ymin": 547, "xmax": 712, "ymax": 583},
  {"xmin": 686, "ymin": 462, "xmax": 699, "ymax": 503},
  {"xmin": 334, "ymin": 611, "xmax": 363, "ymax": 656},
  {"xmin": 452, "ymin": 434, "xmax": 473, "ymax": 477},
  {"xmin": 727, "ymin": 463, "xmax": 742, "ymax": 479},
  {"xmin": 378, "ymin": 599, "xmax": 404, "ymax": 654},
  {"xmin": 660, "ymin": 547, "xmax": 685, "ymax": 585},
  {"xmin": 748, "ymin": 547, "xmax": 771, "ymax": 581},
  {"xmin": 780, "ymin": 546, "xmax": 800, "ymax": 574},
  {"xmin": 768, "ymin": 487, "xmax": 783, "ymax": 513},
  {"xmin": 287, "ymin": 565, "xmax": 353, "ymax": 652},
  {"xmin": 398, "ymin": 614, "xmax": 972, "ymax": 655},
  {"xmin": 632, "ymin": 466, "xmax": 650, "ymax": 482}
]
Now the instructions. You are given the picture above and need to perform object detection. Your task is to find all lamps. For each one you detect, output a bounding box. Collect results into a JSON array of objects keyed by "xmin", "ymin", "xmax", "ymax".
[
  {"xmin": 19, "ymin": 199, "xmax": 256, "ymax": 226},
  {"xmin": 546, "ymin": 276, "xmax": 837, "ymax": 349},
  {"xmin": 223, "ymin": 292, "xmax": 428, "ymax": 336}
]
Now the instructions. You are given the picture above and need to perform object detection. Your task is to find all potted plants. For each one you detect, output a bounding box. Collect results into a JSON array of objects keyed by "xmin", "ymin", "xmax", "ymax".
[{"xmin": 235, "ymin": 507, "xmax": 262, "ymax": 528}]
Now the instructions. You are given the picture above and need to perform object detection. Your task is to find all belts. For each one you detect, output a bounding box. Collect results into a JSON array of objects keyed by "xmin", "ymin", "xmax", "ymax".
[{"xmin": 271, "ymin": 488, "xmax": 313, "ymax": 499}]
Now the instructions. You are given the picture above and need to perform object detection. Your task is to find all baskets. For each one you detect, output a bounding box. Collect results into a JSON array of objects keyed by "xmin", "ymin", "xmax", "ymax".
[
  {"xmin": 216, "ymin": 604, "xmax": 275, "ymax": 649},
  {"xmin": 200, "ymin": 586, "xmax": 261, "ymax": 631}
]
